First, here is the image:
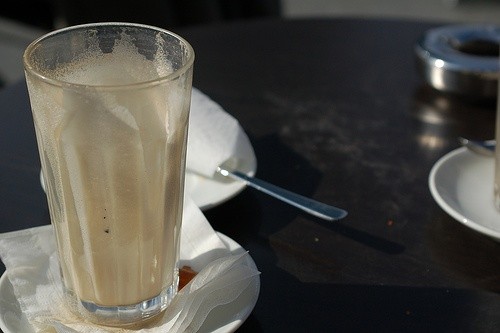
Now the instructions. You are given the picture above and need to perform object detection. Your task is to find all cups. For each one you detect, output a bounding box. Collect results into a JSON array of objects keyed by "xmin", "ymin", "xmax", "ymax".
[{"xmin": 24, "ymin": 23, "xmax": 196, "ymax": 333}]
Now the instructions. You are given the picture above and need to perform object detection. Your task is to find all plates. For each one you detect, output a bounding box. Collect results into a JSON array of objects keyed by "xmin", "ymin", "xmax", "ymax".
[
  {"xmin": 0, "ymin": 219, "xmax": 261, "ymax": 333},
  {"xmin": 428, "ymin": 136, "xmax": 500, "ymax": 241},
  {"xmin": 39, "ymin": 88, "xmax": 255, "ymax": 210}
]
